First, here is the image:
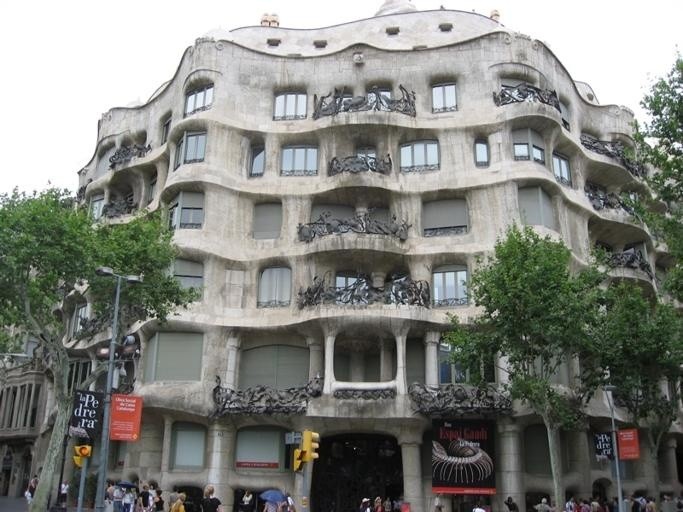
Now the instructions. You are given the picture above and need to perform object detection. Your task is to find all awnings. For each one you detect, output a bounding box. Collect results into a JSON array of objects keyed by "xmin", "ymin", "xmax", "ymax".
[{"xmin": 431, "ymin": 486, "xmax": 496, "ymax": 495}]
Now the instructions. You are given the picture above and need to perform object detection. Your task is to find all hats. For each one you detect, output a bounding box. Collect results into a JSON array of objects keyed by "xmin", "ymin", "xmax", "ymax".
[{"xmin": 361, "ymin": 497, "xmax": 370, "ymax": 502}]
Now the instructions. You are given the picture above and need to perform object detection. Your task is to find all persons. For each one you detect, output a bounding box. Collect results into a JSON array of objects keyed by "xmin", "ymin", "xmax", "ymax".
[
  {"xmin": 24, "ymin": 478, "xmax": 37, "ymax": 503},
  {"xmin": 473, "ymin": 504, "xmax": 485, "ymax": 512},
  {"xmin": 533, "ymin": 497, "xmax": 552, "ymax": 510},
  {"xmin": 33, "ymin": 473, "xmax": 38, "ymax": 486},
  {"xmin": 59, "ymin": 479, "xmax": 69, "ymax": 508},
  {"xmin": 566, "ymin": 492, "xmax": 683, "ymax": 511},
  {"xmin": 360, "ymin": 496, "xmax": 411, "ymax": 512},
  {"xmin": 104, "ymin": 479, "xmax": 223, "ymax": 512},
  {"xmin": 242, "ymin": 488, "xmax": 296, "ymax": 512},
  {"xmin": 503, "ymin": 496, "xmax": 518, "ymax": 510}
]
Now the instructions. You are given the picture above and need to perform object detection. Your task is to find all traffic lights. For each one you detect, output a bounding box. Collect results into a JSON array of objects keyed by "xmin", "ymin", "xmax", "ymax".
[
  {"xmin": 73, "ymin": 445, "xmax": 92, "ymax": 468},
  {"xmin": 293, "ymin": 430, "xmax": 320, "ymax": 472}
]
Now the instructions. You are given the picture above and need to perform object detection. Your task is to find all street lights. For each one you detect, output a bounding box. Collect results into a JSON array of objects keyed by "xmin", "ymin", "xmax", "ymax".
[
  {"xmin": 0, "ymin": 353, "xmax": 29, "ymax": 357},
  {"xmin": 94, "ymin": 266, "xmax": 145, "ymax": 512},
  {"xmin": 601, "ymin": 385, "xmax": 623, "ymax": 512}
]
